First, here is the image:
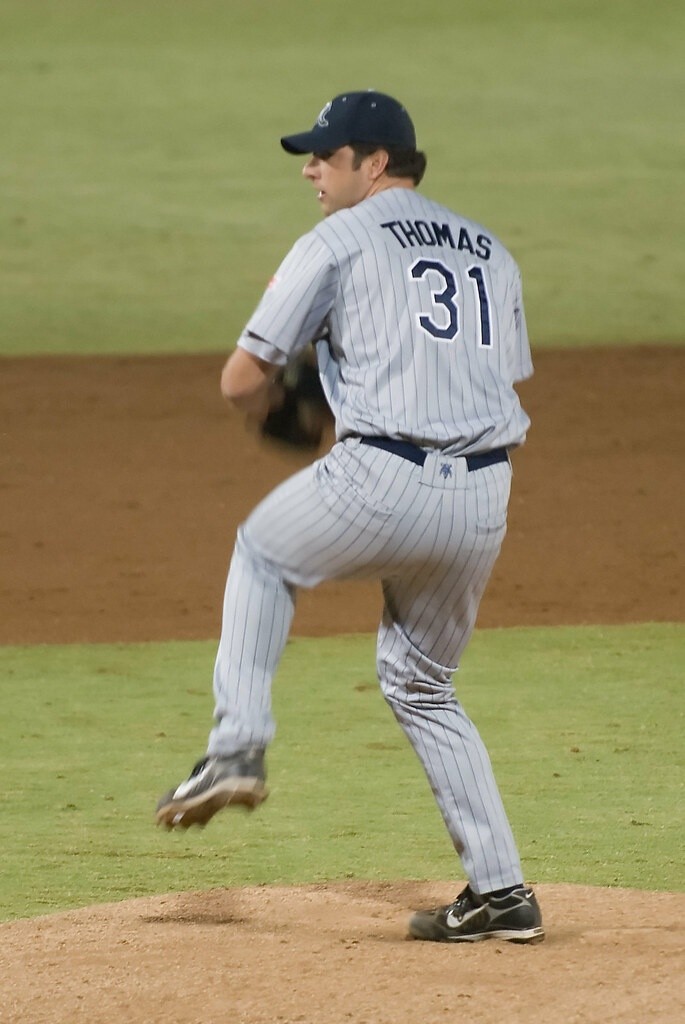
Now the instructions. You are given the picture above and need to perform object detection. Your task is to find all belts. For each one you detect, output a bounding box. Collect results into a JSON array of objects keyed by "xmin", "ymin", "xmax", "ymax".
[{"xmin": 341, "ymin": 434, "xmax": 508, "ymax": 471}]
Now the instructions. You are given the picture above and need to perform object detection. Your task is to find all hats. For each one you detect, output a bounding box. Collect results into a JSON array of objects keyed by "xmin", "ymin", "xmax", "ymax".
[{"xmin": 280, "ymin": 92, "xmax": 416, "ymax": 153}]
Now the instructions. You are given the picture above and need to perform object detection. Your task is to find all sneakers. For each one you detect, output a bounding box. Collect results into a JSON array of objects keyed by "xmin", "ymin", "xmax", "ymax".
[
  {"xmin": 153, "ymin": 750, "xmax": 269, "ymax": 829},
  {"xmin": 409, "ymin": 883, "xmax": 546, "ymax": 945}
]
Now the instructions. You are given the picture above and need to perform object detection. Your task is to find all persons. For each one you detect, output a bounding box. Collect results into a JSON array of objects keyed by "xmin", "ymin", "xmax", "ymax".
[{"xmin": 152, "ymin": 91, "xmax": 547, "ymax": 943}]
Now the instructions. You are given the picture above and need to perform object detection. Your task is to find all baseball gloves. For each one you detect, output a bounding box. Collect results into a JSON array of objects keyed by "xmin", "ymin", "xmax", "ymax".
[{"xmin": 246, "ymin": 350, "xmax": 334, "ymax": 457}]
